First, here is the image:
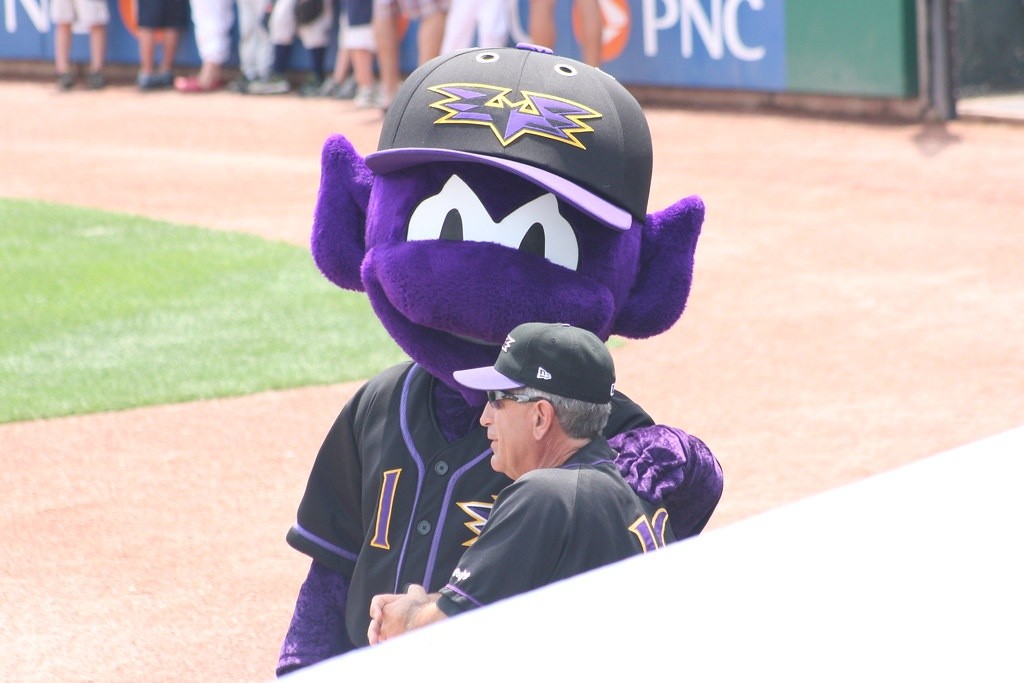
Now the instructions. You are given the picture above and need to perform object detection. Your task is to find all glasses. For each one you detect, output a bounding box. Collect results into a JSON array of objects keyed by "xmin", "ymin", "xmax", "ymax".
[{"xmin": 486, "ymin": 389, "xmax": 554, "ymax": 409}]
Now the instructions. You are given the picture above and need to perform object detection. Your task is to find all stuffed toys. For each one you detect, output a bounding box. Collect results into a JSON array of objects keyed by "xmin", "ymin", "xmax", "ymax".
[{"xmin": 274, "ymin": 41, "xmax": 726, "ymax": 681}]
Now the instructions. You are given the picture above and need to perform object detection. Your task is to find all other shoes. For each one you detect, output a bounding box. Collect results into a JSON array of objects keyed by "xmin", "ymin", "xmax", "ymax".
[
  {"xmin": 139, "ymin": 66, "xmax": 408, "ymax": 115},
  {"xmin": 86, "ymin": 70, "xmax": 104, "ymax": 89},
  {"xmin": 57, "ymin": 71, "xmax": 75, "ymax": 89}
]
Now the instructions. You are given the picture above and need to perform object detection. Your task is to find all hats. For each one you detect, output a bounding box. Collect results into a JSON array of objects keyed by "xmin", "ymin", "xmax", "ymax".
[{"xmin": 452, "ymin": 322, "xmax": 616, "ymax": 404}]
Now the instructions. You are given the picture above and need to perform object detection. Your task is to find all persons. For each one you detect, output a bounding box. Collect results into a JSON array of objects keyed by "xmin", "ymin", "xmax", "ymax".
[
  {"xmin": 368, "ymin": 322, "xmax": 663, "ymax": 645},
  {"xmin": 47, "ymin": 0, "xmax": 610, "ymax": 123}
]
